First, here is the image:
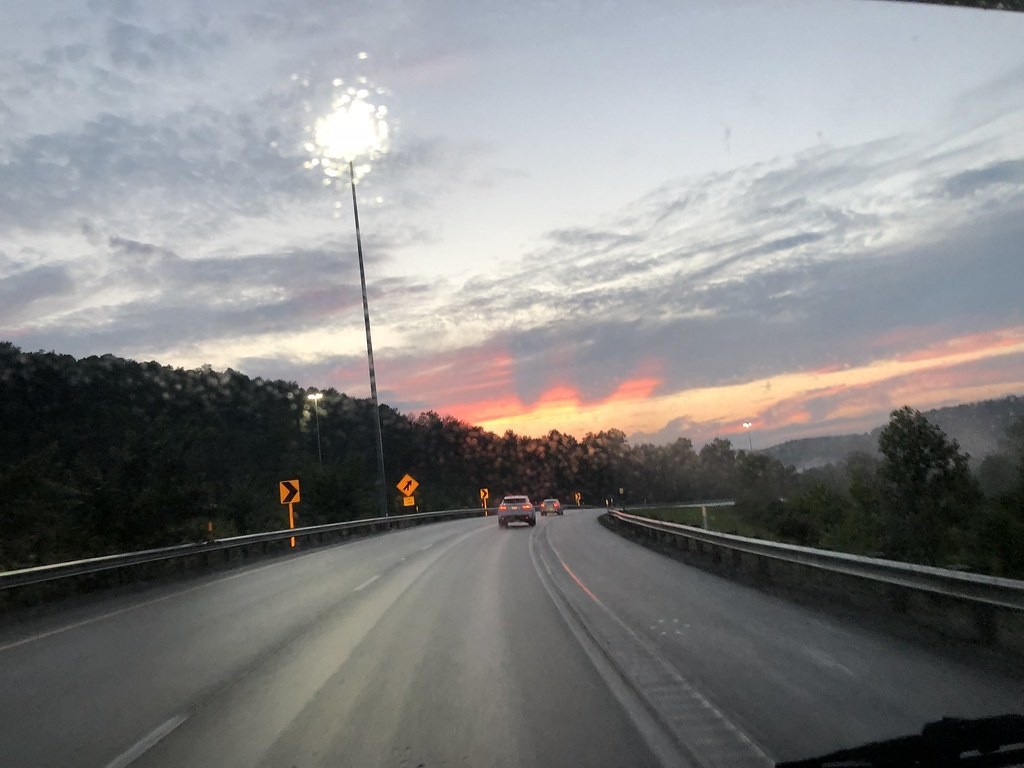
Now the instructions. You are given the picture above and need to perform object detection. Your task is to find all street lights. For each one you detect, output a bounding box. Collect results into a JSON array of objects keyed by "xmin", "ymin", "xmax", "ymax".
[
  {"xmin": 743, "ymin": 421, "xmax": 753, "ymax": 449},
  {"xmin": 309, "ymin": 392, "xmax": 323, "ymax": 469}
]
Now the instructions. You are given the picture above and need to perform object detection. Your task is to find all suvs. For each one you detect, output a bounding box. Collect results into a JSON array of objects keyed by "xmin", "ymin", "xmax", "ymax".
[{"xmin": 497, "ymin": 496, "xmax": 536, "ymax": 529}]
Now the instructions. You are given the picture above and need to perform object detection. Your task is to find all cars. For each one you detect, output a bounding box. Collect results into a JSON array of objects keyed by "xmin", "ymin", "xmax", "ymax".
[{"xmin": 540, "ymin": 499, "xmax": 564, "ymax": 516}]
